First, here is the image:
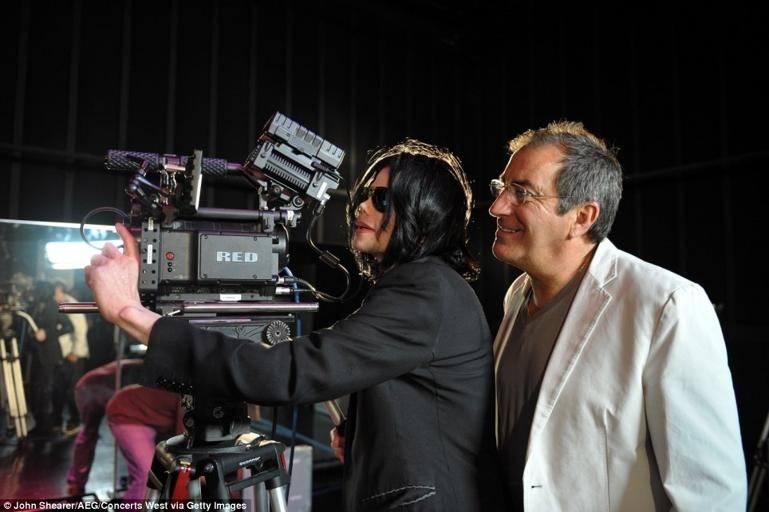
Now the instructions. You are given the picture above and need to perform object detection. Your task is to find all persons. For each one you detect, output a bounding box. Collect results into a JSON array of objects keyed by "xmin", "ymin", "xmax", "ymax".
[
  {"xmin": 488, "ymin": 123, "xmax": 748, "ymax": 512},
  {"xmin": 1, "ymin": 272, "xmax": 90, "ymax": 441},
  {"xmin": 84, "ymin": 124, "xmax": 494, "ymax": 511}
]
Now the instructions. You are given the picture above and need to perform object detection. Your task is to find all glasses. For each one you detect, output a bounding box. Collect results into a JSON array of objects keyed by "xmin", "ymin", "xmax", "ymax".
[
  {"xmin": 355, "ymin": 187, "xmax": 391, "ymax": 212},
  {"xmin": 489, "ymin": 179, "xmax": 567, "ymax": 206}
]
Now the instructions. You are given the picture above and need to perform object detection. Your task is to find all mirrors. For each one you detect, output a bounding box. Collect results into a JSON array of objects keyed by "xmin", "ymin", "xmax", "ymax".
[{"xmin": 0, "ymin": 218, "xmax": 126, "ymax": 512}]
